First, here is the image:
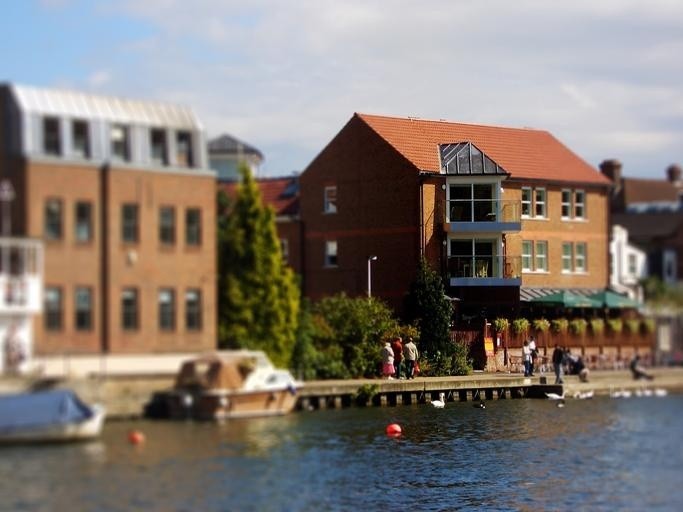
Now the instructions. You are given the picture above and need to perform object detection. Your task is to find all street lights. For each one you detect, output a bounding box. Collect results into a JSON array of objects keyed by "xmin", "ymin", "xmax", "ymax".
[{"xmin": 368, "ymin": 255, "xmax": 377, "ymax": 298}]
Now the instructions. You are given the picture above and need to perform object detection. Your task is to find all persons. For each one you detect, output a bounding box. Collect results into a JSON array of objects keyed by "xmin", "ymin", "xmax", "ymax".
[
  {"xmin": 629, "ymin": 354, "xmax": 641, "ymax": 379},
  {"xmin": 522, "ymin": 335, "xmax": 584, "ymax": 385},
  {"xmin": 380, "ymin": 339, "xmax": 395, "ymax": 379},
  {"xmin": 404, "ymin": 336, "xmax": 419, "ymax": 379},
  {"xmin": 392, "ymin": 336, "xmax": 404, "ymax": 379}
]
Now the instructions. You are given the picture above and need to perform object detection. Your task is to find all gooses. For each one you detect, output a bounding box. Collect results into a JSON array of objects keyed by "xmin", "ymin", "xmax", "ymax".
[
  {"xmin": 544, "ymin": 390, "xmax": 568, "ymax": 400},
  {"xmin": 573, "ymin": 390, "xmax": 594, "ymax": 400},
  {"xmin": 430, "ymin": 393, "xmax": 445, "ymax": 409},
  {"xmin": 609, "ymin": 383, "xmax": 668, "ymax": 398},
  {"xmin": 557, "ymin": 400, "xmax": 565, "ymax": 408},
  {"xmin": 473, "ymin": 399, "xmax": 487, "ymax": 409}
]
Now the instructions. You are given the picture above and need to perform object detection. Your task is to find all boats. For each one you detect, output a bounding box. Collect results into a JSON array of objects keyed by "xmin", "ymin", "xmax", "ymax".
[
  {"xmin": 0, "ymin": 389, "xmax": 105, "ymax": 443},
  {"xmin": 153, "ymin": 350, "xmax": 304, "ymax": 421}
]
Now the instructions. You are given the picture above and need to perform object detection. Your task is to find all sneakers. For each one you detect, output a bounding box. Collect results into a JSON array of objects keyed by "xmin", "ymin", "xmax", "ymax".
[{"xmin": 387, "ymin": 376, "xmax": 412, "ymax": 379}]
